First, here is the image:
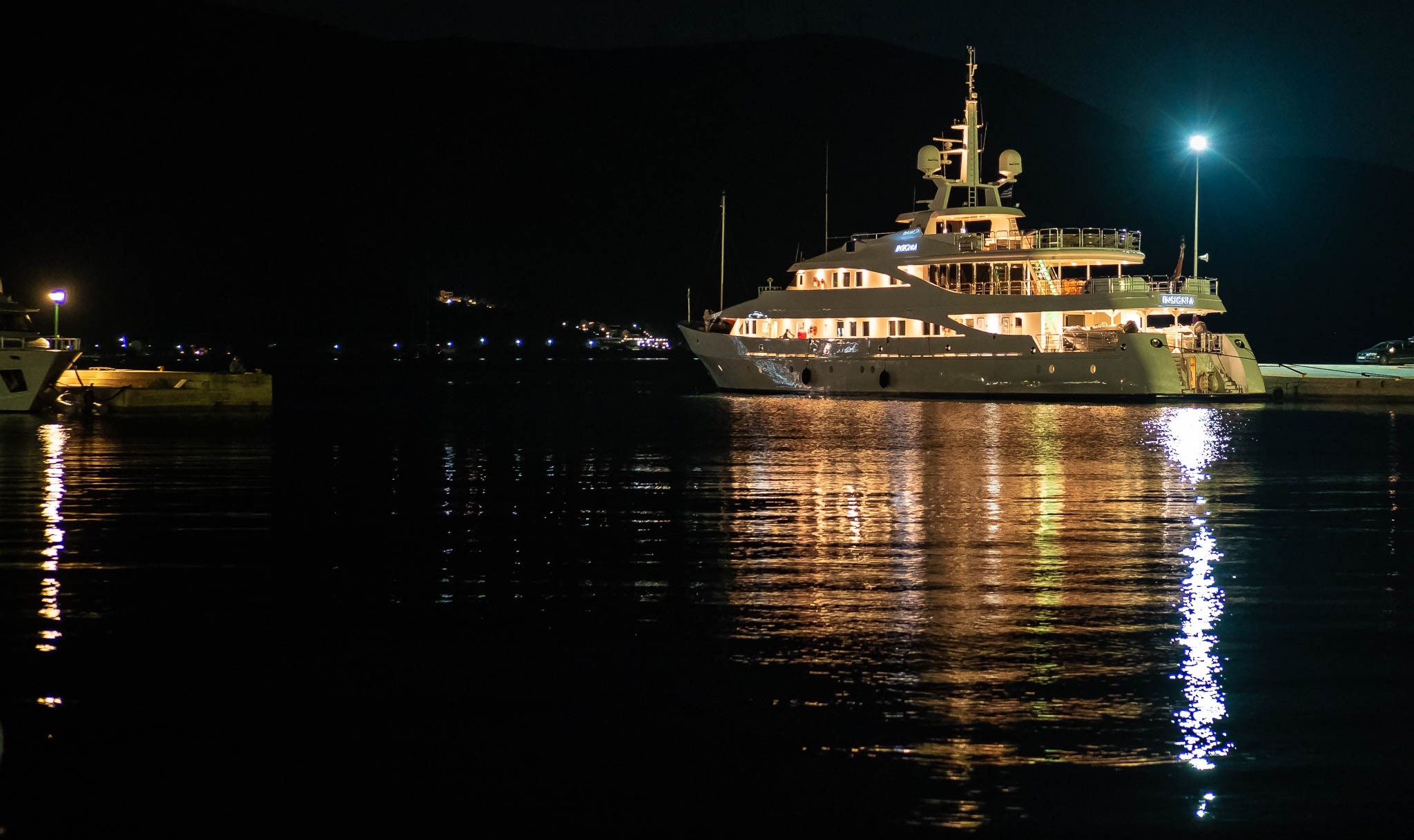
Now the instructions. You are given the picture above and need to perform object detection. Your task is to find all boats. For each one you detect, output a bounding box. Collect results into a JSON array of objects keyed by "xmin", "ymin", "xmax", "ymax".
[
  {"xmin": 674, "ymin": 45, "xmax": 1266, "ymax": 403},
  {"xmin": 0, "ymin": 276, "xmax": 83, "ymax": 412}
]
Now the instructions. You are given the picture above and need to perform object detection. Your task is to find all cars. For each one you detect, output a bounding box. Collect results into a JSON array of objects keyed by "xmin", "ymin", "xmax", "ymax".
[{"xmin": 1356, "ymin": 340, "xmax": 1414, "ymax": 366}]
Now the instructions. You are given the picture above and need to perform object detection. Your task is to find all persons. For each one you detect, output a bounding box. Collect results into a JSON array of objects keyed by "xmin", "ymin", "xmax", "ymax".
[
  {"xmin": 783, "ymin": 329, "xmax": 789, "ymax": 338},
  {"xmin": 798, "ymin": 326, "xmax": 806, "ymax": 339},
  {"xmin": 813, "ymin": 277, "xmax": 816, "ymax": 281},
  {"xmin": 821, "ymin": 279, "xmax": 824, "ymax": 289}
]
